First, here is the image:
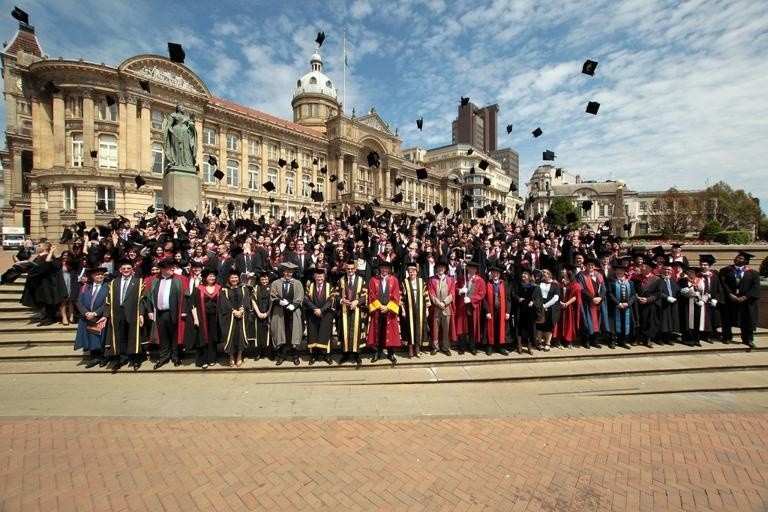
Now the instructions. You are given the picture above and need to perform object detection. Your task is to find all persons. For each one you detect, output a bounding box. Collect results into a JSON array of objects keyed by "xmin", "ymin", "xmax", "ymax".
[
  {"xmin": 12, "ymin": 207, "xmax": 768, "ymax": 368},
  {"xmin": 164, "ymin": 104, "xmax": 197, "ymax": 169}
]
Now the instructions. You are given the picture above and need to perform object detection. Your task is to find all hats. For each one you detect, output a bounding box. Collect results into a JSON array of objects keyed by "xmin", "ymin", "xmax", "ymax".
[
  {"xmin": 263, "ymin": 181, "xmax": 275, "ymax": 191},
  {"xmin": 208, "ymin": 156, "xmax": 224, "ymax": 180},
  {"xmin": 393, "ymin": 178, "xmax": 402, "ymax": 185},
  {"xmin": 533, "ymin": 128, "xmax": 542, "ymax": 137},
  {"xmin": 279, "ymin": 159, "xmax": 344, "ymax": 192},
  {"xmin": 139, "ymin": 81, "xmax": 149, "ymax": 90},
  {"xmin": 461, "ymin": 96, "xmax": 469, "ymax": 108},
  {"xmin": 44, "ymin": 81, "xmax": 60, "ymax": 94},
  {"xmin": 59, "ymin": 191, "xmax": 755, "ymax": 282},
  {"xmin": 507, "ymin": 125, "xmax": 512, "ymax": 134},
  {"xmin": 586, "ymin": 102, "xmax": 600, "ymax": 114},
  {"xmin": 106, "ymin": 95, "xmax": 114, "ymax": 105},
  {"xmin": 543, "ymin": 150, "xmax": 554, "ymax": 160},
  {"xmin": 556, "ymin": 168, "xmax": 560, "ymax": 177},
  {"xmin": 417, "ymin": 117, "xmax": 423, "ymax": 129},
  {"xmin": 316, "ymin": 32, "xmax": 325, "ymax": 47},
  {"xmin": 368, "ymin": 152, "xmax": 380, "ymax": 168},
  {"xmin": 510, "ymin": 181, "xmax": 517, "ymax": 190},
  {"xmin": 90, "ymin": 150, "xmax": 97, "ymax": 159},
  {"xmin": 416, "ymin": 169, "xmax": 426, "ymax": 179},
  {"xmin": 168, "ymin": 43, "xmax": 185, "ymax": 63},
  {"xmin": 467, "ymin": 149, "xmax": 490, "ymax": 186},
  {"xmin": 582, "ymin": 60, "xmax": 598, "ymax": 75},
  {"xmin": 12, "ymin": 7, "xmax": 28, "ymax": 24},
  {"xmin": 135, "ymin": 175, "xmax": 145, "ymax": 189}
]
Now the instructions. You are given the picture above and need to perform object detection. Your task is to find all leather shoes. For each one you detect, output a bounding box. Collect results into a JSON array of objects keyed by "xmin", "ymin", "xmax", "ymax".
[
  {"xmin": 38, "ymin": 316, "xmax": 76, "ymax": 326},
  {"xmin": 85, "ymin": 349, "xmax": 396, "ymax": 374},
  {"xmin": 408, "ymin": 337, "xmax": 754, "ymax": 359}
]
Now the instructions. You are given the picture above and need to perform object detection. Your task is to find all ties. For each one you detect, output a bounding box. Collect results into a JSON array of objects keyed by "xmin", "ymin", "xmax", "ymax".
[
  {"xmin": 589, "ymin": 268, "xmax": 742, "ymax": 300},
  {"xmin": 285, "ymin": 276, "xmax": 445, "ymax": 300},
  {"xmin": 468, "ymin": 278, "xmax": 499, "ymax": 308},
  {"xmin": 89, "ymin": 278, "xmax": 166, "ymax": 312}
]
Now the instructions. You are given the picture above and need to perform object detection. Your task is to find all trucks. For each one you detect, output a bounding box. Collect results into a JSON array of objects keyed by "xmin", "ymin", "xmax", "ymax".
[{"xmin": 0, "ymin": 226, "xmax": 27, "ymax": 250}]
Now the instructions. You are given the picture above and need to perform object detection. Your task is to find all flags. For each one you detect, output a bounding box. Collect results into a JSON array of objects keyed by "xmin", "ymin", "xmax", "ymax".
[{"xmin": 345, "ymin": 48, "xmax": 348, "ymax": 66}]
